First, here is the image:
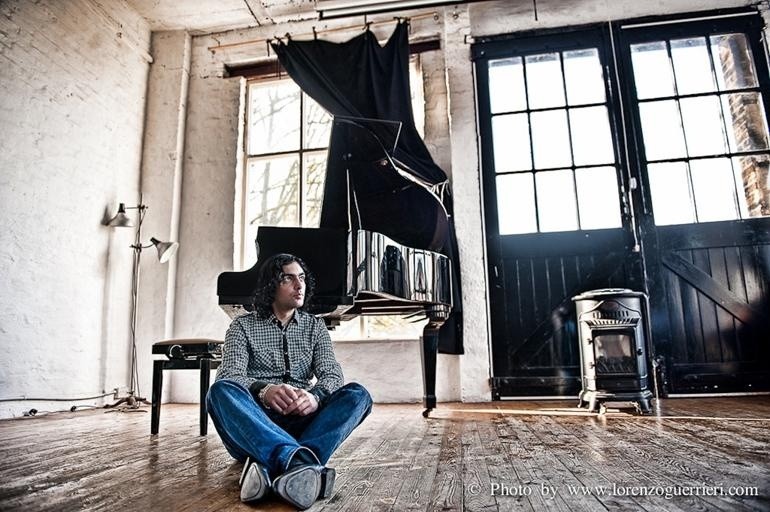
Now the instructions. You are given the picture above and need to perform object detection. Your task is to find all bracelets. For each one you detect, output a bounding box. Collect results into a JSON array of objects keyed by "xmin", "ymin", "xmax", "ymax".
[
  {"xmin": 257, "ymin": 382, "xmax": 276, "ymax": 410},
  {"xmin": 312, "ymin": 393, "xmax": 322, "ymax": 403}
]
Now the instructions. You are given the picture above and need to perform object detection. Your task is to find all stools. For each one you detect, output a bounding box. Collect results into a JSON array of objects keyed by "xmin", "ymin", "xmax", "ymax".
[{"xmin": 150, "ymin": 338, "xmax": 225, "ymax": 435}]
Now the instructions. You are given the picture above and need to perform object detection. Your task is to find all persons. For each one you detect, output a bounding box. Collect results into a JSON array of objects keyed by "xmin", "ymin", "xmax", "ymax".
[{"xmin": 203, "ymin": 253, "xmax": 374, "ymax": 510}]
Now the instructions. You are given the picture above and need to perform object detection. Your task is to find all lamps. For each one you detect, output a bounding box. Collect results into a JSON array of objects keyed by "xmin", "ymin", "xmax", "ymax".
[{"xmin": 107, "ymin": 203, "xmax": 181, "ymax": 408}]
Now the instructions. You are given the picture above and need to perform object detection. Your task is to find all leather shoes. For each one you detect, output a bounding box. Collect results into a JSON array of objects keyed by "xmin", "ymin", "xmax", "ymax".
[
  {"xmin": 237, "ymin": 456, "xmax": 272, "ymax": 503},
  {"xmin": 273, "ymin": 463, "xmax": 336, "ymax": 511}
]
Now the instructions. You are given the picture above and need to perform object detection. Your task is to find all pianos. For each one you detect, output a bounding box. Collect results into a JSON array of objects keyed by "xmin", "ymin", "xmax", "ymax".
[{"xmin": 216, "ymin": 228, "xmax": 454, "ymax": 417}]
